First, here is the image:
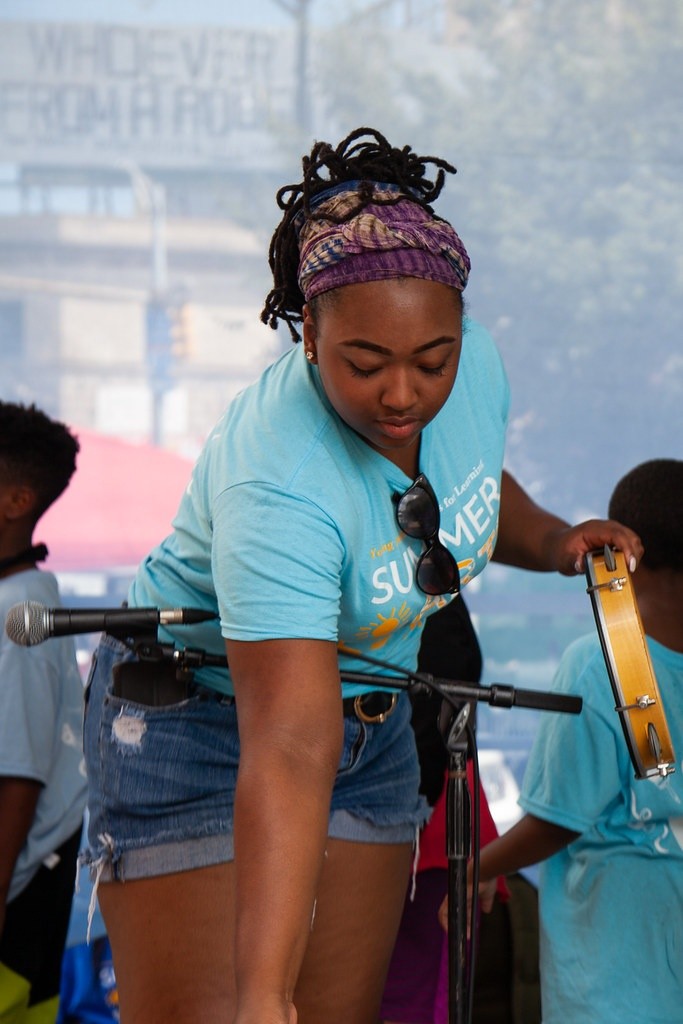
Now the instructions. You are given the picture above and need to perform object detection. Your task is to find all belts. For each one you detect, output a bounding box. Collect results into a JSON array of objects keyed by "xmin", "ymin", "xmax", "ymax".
[{"xmin": 214, "ymin": 687, "xmax": 399, "ymax": 723}]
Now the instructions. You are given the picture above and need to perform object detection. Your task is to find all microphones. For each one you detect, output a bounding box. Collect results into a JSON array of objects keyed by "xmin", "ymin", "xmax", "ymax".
[{"xmin": 5, "ymin": 600, "xmax": 218, "ymax": 647}]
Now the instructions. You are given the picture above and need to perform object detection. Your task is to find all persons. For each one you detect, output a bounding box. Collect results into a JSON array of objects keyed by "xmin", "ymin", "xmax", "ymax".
[
  {"xmin": 0, "ymin": 402, "xmax": 88, "ymax": 1008},
  {"xmin": 82, "ymin": 129, "xmax": 642, "ymax": 1024},
  {"xmin": 437, "ymin": 457, "xmax": 683, "ymax": 1024}
]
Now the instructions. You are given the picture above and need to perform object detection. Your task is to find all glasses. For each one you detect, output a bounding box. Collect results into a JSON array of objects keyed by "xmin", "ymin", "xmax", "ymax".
[{"xmin": 391, "ymin": 474, "xmax": 462, "ymax": 597}]
